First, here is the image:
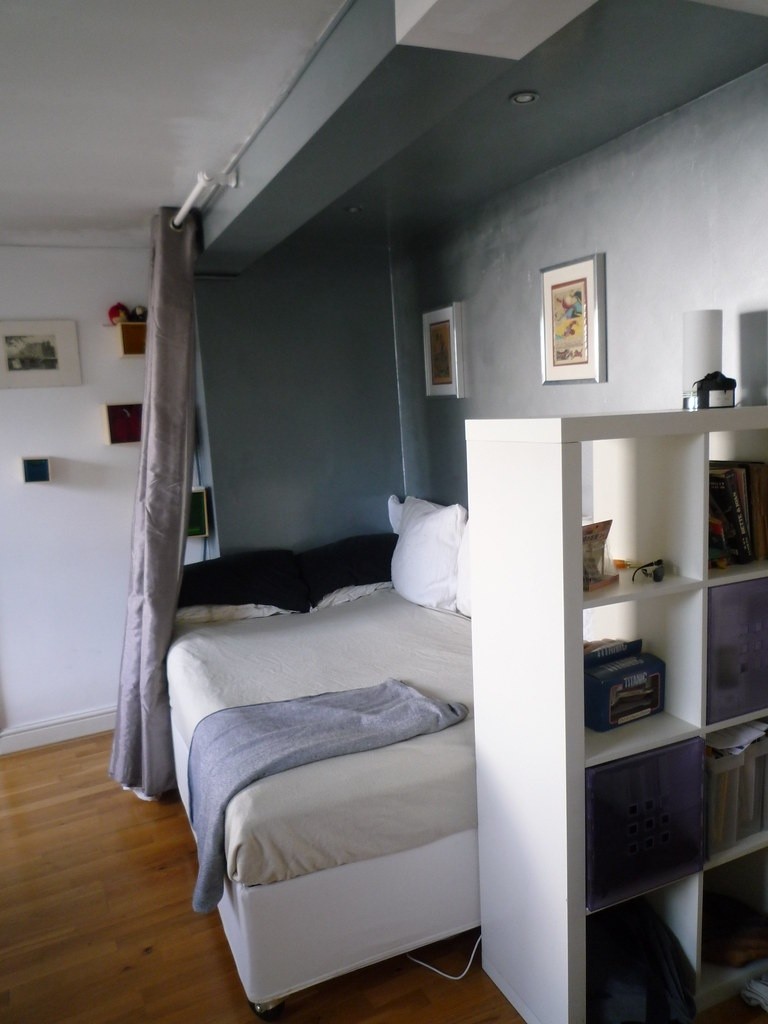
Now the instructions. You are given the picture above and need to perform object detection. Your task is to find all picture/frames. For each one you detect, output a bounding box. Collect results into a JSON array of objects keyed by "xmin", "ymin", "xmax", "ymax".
[
  {"xmin": 538, "ymin": 252, "xmax": 607, "ymax": 386},
  {"xmin": 420, "ymin": 300, "xmax": 465, "ymax": 400},
  {"xmin": 1, "ymin": 319, "xmax": 82, "ymax": 388}
]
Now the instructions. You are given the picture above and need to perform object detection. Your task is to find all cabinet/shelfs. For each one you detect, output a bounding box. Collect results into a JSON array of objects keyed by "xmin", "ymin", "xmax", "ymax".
[{"xmin": 462, "ymin": 406, "xmax": 767, "ymax": 1024}]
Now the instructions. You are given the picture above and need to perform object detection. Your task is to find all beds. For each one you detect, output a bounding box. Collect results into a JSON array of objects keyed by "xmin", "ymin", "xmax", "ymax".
[{"xmin": 162, "ymin": 589, "xmax": 485, "ymax": 1014}]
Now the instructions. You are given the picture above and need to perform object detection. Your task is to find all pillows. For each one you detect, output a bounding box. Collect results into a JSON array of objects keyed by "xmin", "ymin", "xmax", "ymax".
[
  {"xmin": 176, "ymin": 547, "xmax": 312, "ymax": 613},
  {"xmin": 452, "ymin": 516, "xmax": 471, "ymax": 618},
  {"xmin": 173, "ymin": 603, "xmax": 298, "ymax": 625},
  {"xmin": 297, "ymin": 533, "xmax": 400, "ymax": 608},
  {"xmin": 309, "ymin": 580, "xmax": 393, "ymax": 613},
  {"xmin": 387, "ymin": 495, "xmax": 469, "ymax": 614}
]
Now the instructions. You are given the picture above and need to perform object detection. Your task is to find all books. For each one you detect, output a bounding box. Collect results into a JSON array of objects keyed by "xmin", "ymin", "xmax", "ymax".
[
  {"xmin": 709, "ymin": 462, "xmax": 768, "ymax": 569},
  {"xmin": 706, "ymin": 724, "xmax": 768, "ymax": 852}
]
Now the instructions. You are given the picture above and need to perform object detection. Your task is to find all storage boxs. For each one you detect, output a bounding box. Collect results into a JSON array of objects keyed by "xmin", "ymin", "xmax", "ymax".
[
  {"xmin": 705, "ymin": 735, "xmax": 767, "ymax": 854},
  {"xmin": 583, "ymin": 638, "xmax": 667, "ymax": 732},
  {"xmin": 584, "ymin": 736, "xmax": 706, "ymax": 914},
  {"xmin": 706, "ymin": 578, "xmax": 768, "ymax": 725}
]
